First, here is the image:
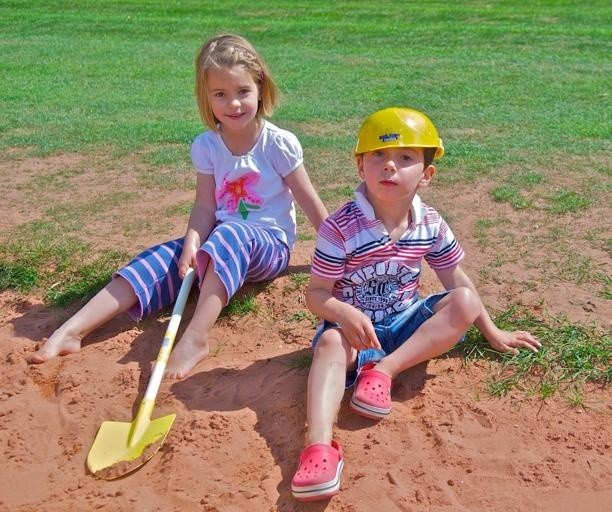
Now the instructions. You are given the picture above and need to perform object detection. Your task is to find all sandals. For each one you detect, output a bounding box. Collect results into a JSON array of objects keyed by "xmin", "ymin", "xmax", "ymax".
[
  {"xmin": 292, "ymin": 439, "xmax": 345, "ymax": 502},
  {"xmin": 351, "ymin": 361, "xmax": 393, "ymax": 419}
]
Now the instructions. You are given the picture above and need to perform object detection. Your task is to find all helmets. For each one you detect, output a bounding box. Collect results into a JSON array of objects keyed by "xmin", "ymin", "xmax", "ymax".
[{"xmin": 354, "ymin": 107, "xmax": 444, "ymax": 160}]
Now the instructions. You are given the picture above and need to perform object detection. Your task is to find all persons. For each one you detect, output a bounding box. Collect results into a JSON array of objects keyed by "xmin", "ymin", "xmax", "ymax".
[
  {"xmin": 290, "ymin": 108, "xmax": 542, "ymax": 503},
  {"xmin": 26, "ymin": 34, "xmax": 330, "ymax": 380}
]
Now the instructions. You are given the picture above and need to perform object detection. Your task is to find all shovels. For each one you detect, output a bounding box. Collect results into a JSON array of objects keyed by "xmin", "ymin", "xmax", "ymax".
[{"xmin": 86, "ymin": 266, "xmax": 195, "ymax": 480}]
